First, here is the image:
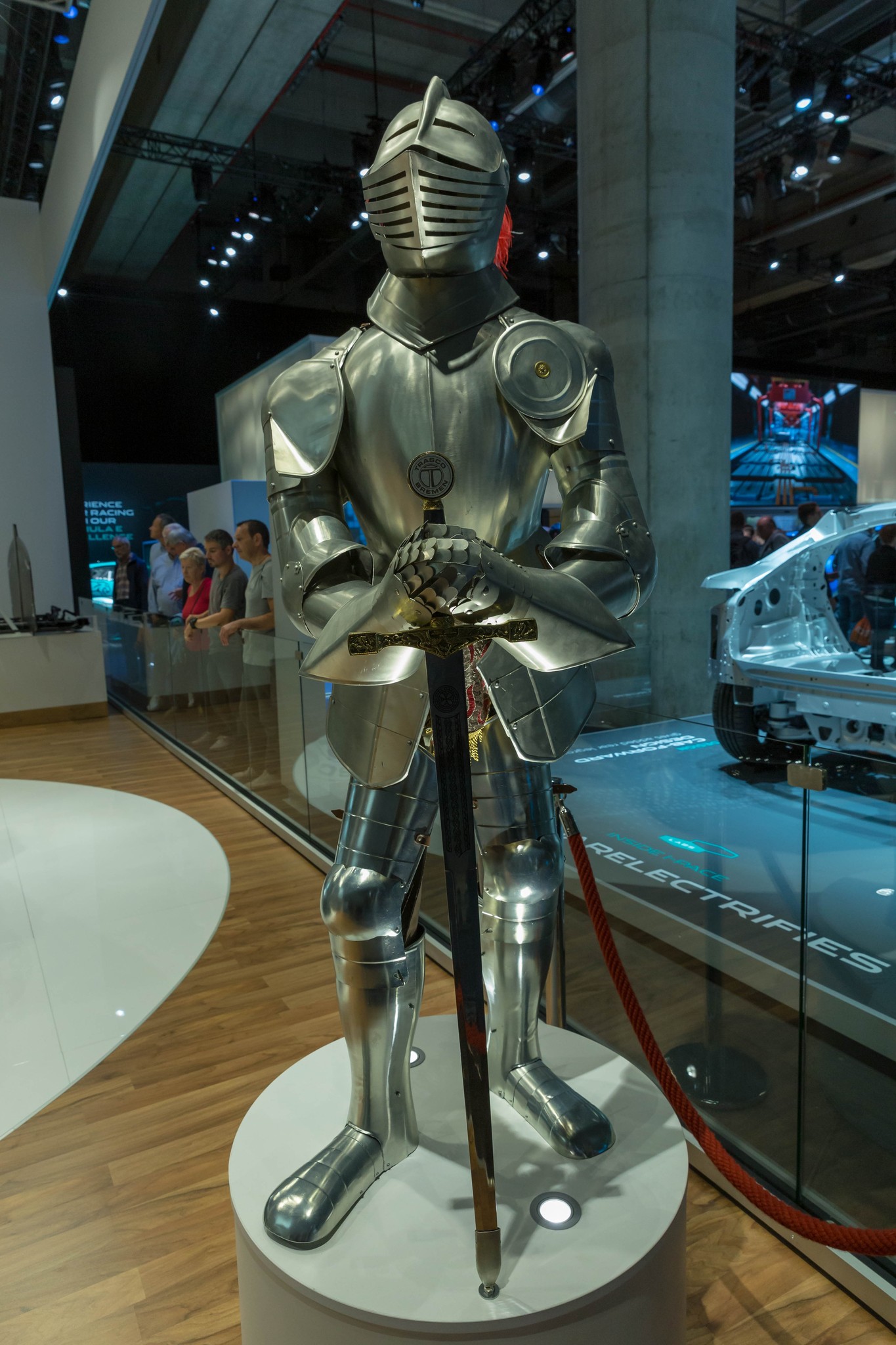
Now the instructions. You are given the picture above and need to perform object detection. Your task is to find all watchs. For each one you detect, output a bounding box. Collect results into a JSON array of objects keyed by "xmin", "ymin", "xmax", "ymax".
[{"xmin": 189, "ymin": 617, "xmax": 197, "ymax": 629}]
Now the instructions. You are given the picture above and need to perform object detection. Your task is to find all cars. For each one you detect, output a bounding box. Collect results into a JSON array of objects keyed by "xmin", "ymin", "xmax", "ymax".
[{"xmin": 699, "ymin": 502, "xmax": 896, "ymax": 780}]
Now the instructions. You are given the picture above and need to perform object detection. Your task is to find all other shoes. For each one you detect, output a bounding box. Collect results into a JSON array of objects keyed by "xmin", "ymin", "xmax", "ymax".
[
  {"xmin": 209, "ymin": 735, "xmax": 236, "ymax": 752},
  {"xmin": 245, "ymin": 769, "xmax": 281, "ymax": 791},
  {"xmin": 191, "ymin": 732, "xmax": 216, "ymax": 747},
  {"xmin": 231, "ymin": 766, "xmax": 260, "ymax": 784},
  {"xmin": 146, "ymin": 694, "xmax": 163, "ymax": 710}
]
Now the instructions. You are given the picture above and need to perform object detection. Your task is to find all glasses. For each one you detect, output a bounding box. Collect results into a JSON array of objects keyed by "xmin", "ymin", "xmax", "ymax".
[{"xmin": 111, "ymin": 542, "xmax": 126, "ymax": 551}]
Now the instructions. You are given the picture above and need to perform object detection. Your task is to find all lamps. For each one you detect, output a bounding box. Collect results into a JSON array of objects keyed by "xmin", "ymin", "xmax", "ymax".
[
  {"xmin": 27, "ymin": 0, "xmax": 575, "ymax": 320},
  {"xmin": 738, "ymin": 51, "xmax": 863, "ymax": 282}
]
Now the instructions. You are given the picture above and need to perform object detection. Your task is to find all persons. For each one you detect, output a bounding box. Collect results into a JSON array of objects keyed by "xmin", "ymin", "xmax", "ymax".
[
  {"xmin": 112, "ymin": 514, "xmax": 282, "ymax": 791},
  {"xmin": 730, "ymin": 502, "xmax": 896, "ymax": 672},
  {"xmin": 263, "ymin": 75, "xmax": 657, "ymax": 1250}
]
